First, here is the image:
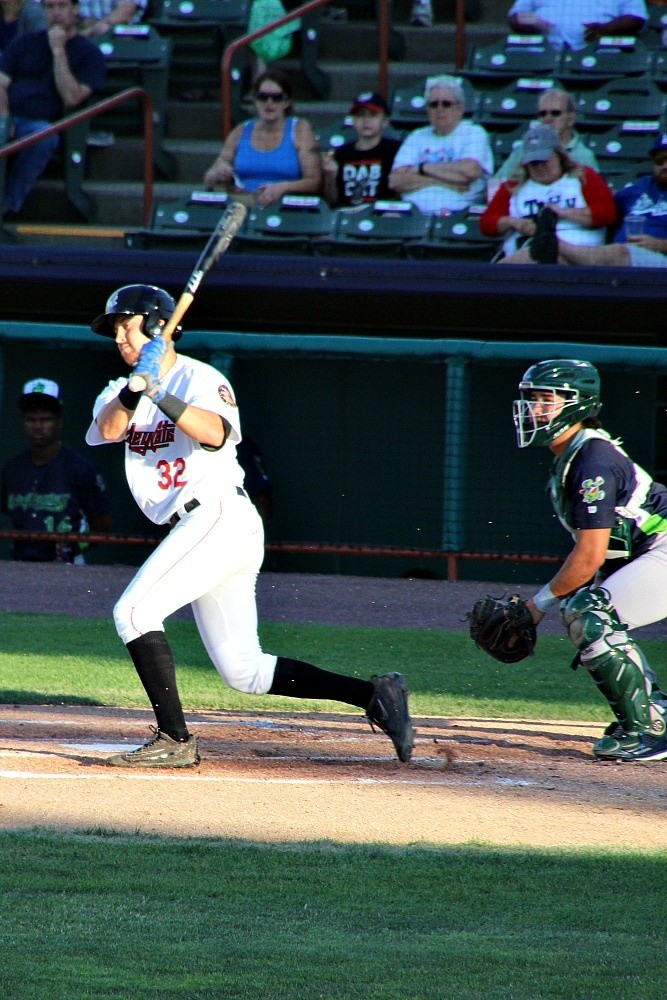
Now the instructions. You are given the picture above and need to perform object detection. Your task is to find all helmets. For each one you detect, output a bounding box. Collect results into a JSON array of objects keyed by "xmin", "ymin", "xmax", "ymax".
[
  {"xmin": 515, "ymin": 358, "xmax": 603, "ymax": 446},
  {"xmin": 92, "ymin": 284, "xmax": 183, "ymax": 341}
]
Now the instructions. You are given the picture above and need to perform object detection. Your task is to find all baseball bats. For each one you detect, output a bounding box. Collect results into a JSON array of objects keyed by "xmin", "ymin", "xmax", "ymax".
[{"xmin": 127, "ymin": 202, "xmax": 245, "ymax": 393}]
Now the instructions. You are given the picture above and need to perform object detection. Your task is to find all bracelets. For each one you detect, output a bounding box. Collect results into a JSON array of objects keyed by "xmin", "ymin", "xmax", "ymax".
[
  {"xmin": 417, "ymin": 162, "xmax": 427, "ymax": 178},
  {"xmin": 533, "ymin": 583, "xmax": 558, "ymax": 612},
  {"xmin": 157, "ymin": 392, "xmax": 187, "ymax": 423},
  {"xmin": 118, "ymin": 384, "xmax": 143, "ymax": 412}
]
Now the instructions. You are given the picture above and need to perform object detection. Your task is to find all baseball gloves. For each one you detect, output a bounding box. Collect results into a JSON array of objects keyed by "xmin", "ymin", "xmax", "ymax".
[{"xmin": 467, "ymin": 594, "xmax": 536, "ymax": 664}]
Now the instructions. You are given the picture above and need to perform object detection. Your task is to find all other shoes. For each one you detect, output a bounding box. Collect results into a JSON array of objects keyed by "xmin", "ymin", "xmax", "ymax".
[{"xmin": 530, "ymin": 208, "xmax": 558, "ymax": 263}]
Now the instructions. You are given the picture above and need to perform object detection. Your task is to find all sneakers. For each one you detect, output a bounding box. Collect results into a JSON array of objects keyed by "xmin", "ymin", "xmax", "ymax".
[
  {"xmin": 605, "ymin": 732, "xmax": 667, "ymax": 761},
  {"xmin": 106, "ymin": 724, "xmax": 200, "ymax": 767},
  {"xmin": 367, "ymin": 672, "xmax": 417, "ymax": 762}
]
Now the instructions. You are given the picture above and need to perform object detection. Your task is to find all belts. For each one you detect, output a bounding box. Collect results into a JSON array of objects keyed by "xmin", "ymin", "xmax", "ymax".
[{"xmin": 164, "ymin": 487, "xmax": 246, "ymax": 533}]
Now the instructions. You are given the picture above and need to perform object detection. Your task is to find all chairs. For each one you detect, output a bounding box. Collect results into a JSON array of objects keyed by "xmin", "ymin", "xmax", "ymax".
[
  {"xmin": 123, "ymin": 193, "xmax": 496, "ymax": 260},
  {"xmin": 58, "ymin": 105, "xmax": 97, "ymax": 221},
  {"xmin": 147, "ymin": 0, "xmax": 258, "ymax": 126},
  {"xmin": 315, "ymin": 41, "xmax": 667, "ymax": 191},
  {"xmin": 293, "ymin": 7, "xmax": 332, "ymax": 100},
  {"xmin": 97, "ymin": 25, "xmax": 175, "ymax": 177}
]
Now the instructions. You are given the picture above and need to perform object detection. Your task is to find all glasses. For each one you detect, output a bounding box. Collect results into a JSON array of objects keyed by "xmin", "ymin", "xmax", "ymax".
[
  {"xmin": 257, "ymin": 93, "xmax": 284, "ymax": 102},
  {"xmin": 427, "ymin": 101, "xmax": 459, "ymax": 108},
  {"xmin": 655, "ymin": 158, "xmax": 667, "ymax": 165},
  {"xmin": 538, "ymin": 110, "xmax": 571, "ymax": 117}
]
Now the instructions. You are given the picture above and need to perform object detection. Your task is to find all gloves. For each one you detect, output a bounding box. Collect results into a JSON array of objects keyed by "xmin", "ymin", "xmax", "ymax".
[{"xmin": 129, "ymin": 335, "xmax": 168, "ymax": 404}]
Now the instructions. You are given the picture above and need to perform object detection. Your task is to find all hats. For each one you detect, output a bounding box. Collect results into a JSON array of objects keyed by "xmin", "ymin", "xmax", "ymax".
[
  {"xmin": 521, "ymin": 126, "xmax": 561, "ymax": 166},
  {"xmin": 22, "ymin": 378, "xmax": 64, "ymax": 406},
  {"xmin": 349, "ymin": 92, "xmax": 388, "ymax": 114},
  {"xmin": 649, "ymin": 134, "xmax": 667, "ymax": 153}
]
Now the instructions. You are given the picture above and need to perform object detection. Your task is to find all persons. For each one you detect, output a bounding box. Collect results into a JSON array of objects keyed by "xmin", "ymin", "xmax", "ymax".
[
  {"xmin": 205, "ymin": 73, "xmax": 322, "ymax": 204},
  {"xmin": 84, "ymin": 282, "xmax": 416, "ymax": 768},
  {"xmin": 508, "ymin": 1, "xmax": 651, "ymax": 52},
  {"xmin": 0, "ymin": 377, "xmax": 107, "ymax": 563},
  {"xmin": 494, "ymin": 87, "xmax": 667, "ymax": 266},
  {"xmin": 511, "ymin": 360, "xmax": 667, "ymax": 763},
  {"xmin": 322, "ymin": 93, "xmax": 403, "ymax": 208},
  {"xmin": 389, "ymin": 75, "xmax": 494, "ymax": 214},
  {"xmin": 0, "ymin": 0, "xmax": 147, "ymax": 215}
]
